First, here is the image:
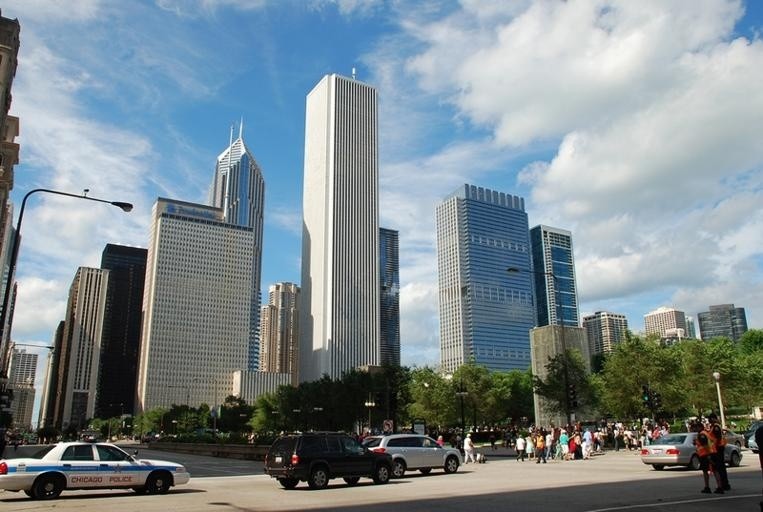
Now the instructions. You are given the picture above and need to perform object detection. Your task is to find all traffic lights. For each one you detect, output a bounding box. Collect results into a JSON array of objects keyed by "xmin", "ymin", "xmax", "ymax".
[
  {"xmin": 641, "ymin": 385, "xmax": 661, "ymax": 409},
  {"xmin": 568, "ymin": 384, "xmax": 577, "ymax": 408}
]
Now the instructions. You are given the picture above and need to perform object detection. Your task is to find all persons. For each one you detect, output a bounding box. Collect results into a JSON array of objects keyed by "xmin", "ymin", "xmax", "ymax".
[
  {"xmin": 687, "ymin": 416, "xmax": 731, "ymax": 494},
  {"xmin": 350, "ymin": 414, "xmax": 731, "ymax": 465},
  {"xmin": 755, "ymin": 426, "xmax": 763, "ymax": 479},
  {"xmin": 0, "ymin": 437, "xmax": 20, "ymax": 459}
]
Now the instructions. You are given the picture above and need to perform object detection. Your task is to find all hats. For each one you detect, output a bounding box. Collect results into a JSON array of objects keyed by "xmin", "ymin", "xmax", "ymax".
[{"xmin": 706, "ymin": 413, "xmax": 717, "ymax": 419}]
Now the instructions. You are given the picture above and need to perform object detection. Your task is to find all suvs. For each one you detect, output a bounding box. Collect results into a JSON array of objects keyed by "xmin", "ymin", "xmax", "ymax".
[{"xmin": 264, "ymin": 431, "xmax": 394, "ymax": 490}]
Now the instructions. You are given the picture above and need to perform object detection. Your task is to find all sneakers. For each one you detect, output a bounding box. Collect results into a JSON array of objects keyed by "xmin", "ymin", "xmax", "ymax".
[{"xmin": 701, "ymin": 485, "xmax": 730, "ymax": 495}]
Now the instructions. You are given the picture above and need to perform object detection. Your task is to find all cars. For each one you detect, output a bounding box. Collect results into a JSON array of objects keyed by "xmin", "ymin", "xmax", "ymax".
[
  {"xmin": 642, "ymin": 429, "xmax": 744, "ymax": 470},
  {"xmin": 1, "ymin": 442, "xmax": 190, "ymax": 502},
  {"xmin": 744, "ymin": 421, "xmax": 763, "ymax": 452},
  {"xmin": 360, "ymin": 433, "xmax": 462, "ymax": 479}
]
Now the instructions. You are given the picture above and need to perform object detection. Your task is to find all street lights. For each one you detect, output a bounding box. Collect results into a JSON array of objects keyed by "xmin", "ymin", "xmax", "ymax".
[
  {"xmin": 454, "ymin": 379, "xmax": 468, "ymax": 431},
  {"xmin": 1, "ymin": 187, "xmax": 135, "ymax": 375},
  {"xmin": 169, "ymin": 377, "xmax": 217, "ymax": 442},
  {"xmin": 713, "ymin": 371, "xmax": 724, "ymax": 428},
  {"xmin": 507, "ymin": 267, "xmax": 572, "ymax": 425},
  {"xmin": 364, "ymin": 392, "xmax": 376, "ymax": 429}
]
